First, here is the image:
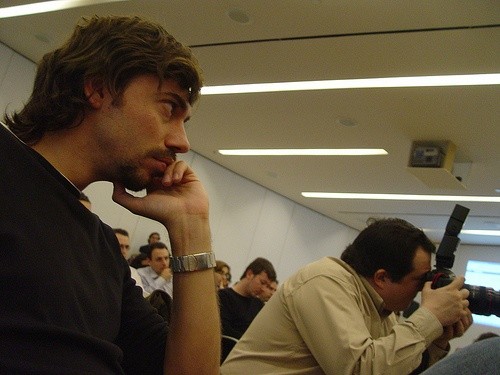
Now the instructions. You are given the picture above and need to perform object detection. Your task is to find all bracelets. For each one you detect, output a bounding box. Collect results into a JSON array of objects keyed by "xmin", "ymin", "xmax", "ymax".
[{"xmin": 169, "ymin": 252, "xmax": 216, "ymax": 273}]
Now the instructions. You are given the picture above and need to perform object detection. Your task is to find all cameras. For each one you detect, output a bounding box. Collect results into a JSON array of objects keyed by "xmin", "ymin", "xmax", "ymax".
[{"xmin": 422, "ymin": 204, "xmax": 500, "ymax": 318}]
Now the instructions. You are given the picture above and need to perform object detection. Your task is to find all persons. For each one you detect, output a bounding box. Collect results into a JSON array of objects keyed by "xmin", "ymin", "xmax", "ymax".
[
  {"xmin": 77, "ymin": 192, "xmax": 173, "ymax": 326},
  {"xmin": 0, "ymin": 14, "xmax": 222, "ymax": 375},
  {"xmin": 214, "ymin": 216, "xmax": 500, "ymax": 375}
]
